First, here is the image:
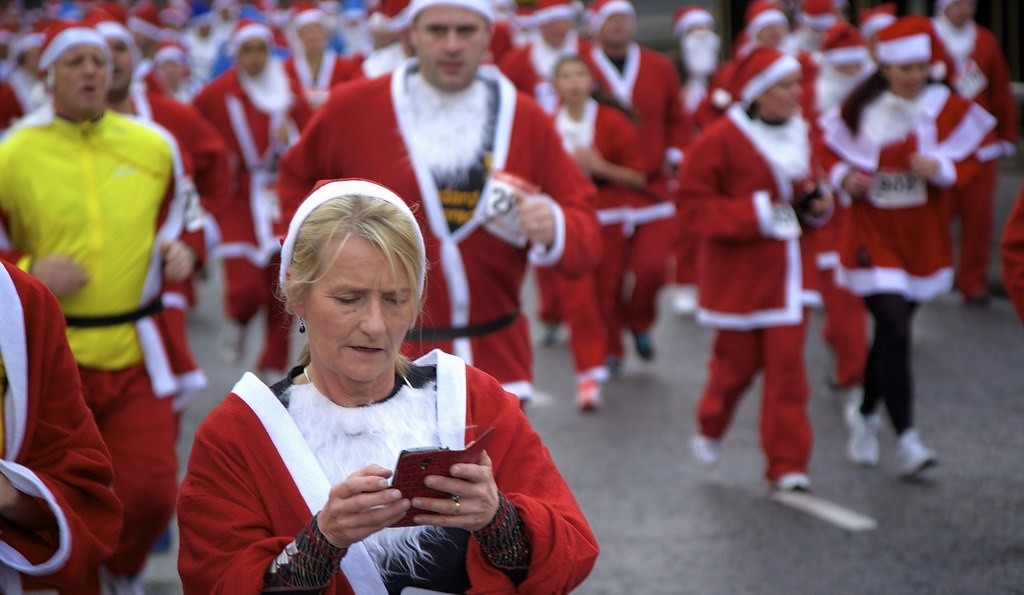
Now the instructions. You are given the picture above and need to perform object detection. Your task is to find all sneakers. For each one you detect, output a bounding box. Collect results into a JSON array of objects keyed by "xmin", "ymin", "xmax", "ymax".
[
  {"xmin": 850, "ymin": 412, "xmax": 881, "ymax": 465},
  {"xmin": 897, "ymin": 432, "xmax": 937, "ymax": 477}
]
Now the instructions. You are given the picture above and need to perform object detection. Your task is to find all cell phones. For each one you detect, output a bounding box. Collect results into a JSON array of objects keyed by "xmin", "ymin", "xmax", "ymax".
[{"xmin": 388, "ymin": 428, "xmax": 494, "ymax": 527}]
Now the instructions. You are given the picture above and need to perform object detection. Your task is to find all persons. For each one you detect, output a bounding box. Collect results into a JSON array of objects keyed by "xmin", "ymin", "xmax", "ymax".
[
  {"xmin": 787, "ymin": 0, "xmax": 1024, "ymax": 394},
  {"xmin": 272, "ymin": 1, "xmax": 602, "ymax": 409},
  {"xmin": 818, "ymin": 18, "xmax": 1000, "ymax": 470},
  {"xmin": 175, "ymin": 175, "xmax": 601, "ymax": 595},
  {"xmin": 0, "ymin": 258, "xmax": 126, "ymax": 595},
  {"xmin": 1, "ymin": 0, "xmax": 411, "ymax": 384},
  {"xmin": 673, "ymin": 43, "xmax": 834, "ymax": 492},
  {"xmin": 0, "ymin": 19, "xmax": 213, "ymax": 595},
  {"xmin": 485, "ymin": 0, "xmax": 787, "ymax": 412}
]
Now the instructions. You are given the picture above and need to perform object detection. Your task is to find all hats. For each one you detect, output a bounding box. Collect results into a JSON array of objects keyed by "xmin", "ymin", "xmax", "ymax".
[
  {"xmin": 279, "ymin": 177, "xmax": 428, "ymax": 305},
  {"xmin": 0, "ymin": 0, "xmax": 979, "ymax": 114}
]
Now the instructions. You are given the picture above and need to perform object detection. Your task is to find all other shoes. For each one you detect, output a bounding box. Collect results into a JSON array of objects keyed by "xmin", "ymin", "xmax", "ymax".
[
  {"xmin": 844, "ymin": 385, "xmax": 867, "ymax": 426},
  {"xmin": 695, "ymin": 427, "xmax": 720, "ymax": 465},
  {"xmin": 780, "ymin": 471, "xmax": 811, "ymax": 491},
  {"xmin": 580, "ymin": 378, "xmax": 604, "ymax": 410},
  {"xmin": 635, "ymin": 326, "xmax": 653, "ymax": 360}
]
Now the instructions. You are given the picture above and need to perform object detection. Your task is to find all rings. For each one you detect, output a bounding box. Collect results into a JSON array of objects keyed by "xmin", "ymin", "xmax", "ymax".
[{"xmin": 453, "ymin": 499, "xmax": 460, "ymax": 514}]
